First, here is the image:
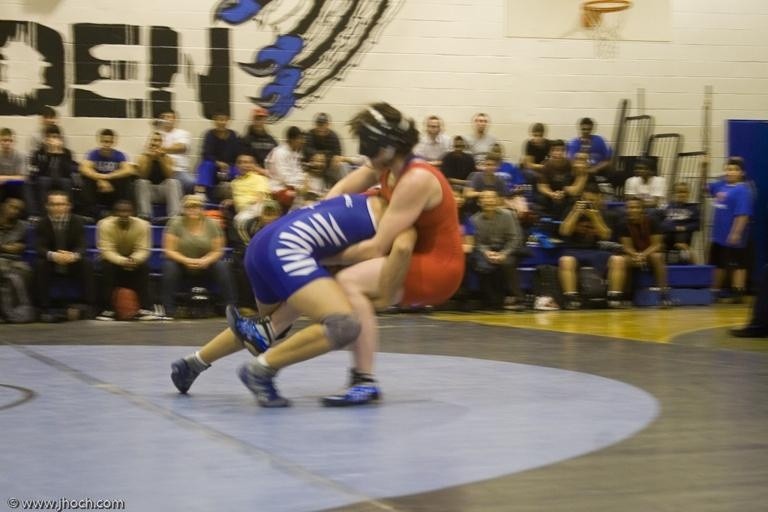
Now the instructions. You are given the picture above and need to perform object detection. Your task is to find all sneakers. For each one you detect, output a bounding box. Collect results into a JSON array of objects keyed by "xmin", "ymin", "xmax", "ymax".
[
  {"xmin": 226, "ymin": 303, "xmax": 270, "ymax": 356},
  {"xmin": 606, "ymin": 293, "xmax": 623, "ymax": 308},
  {"xmin": 95, "ymin": 308, "xmax": 158, "ymax": 321},
  {"xmin": 43, "ymin": 307, "xmax": 91, "ymax": 321},
  {"xmin": 502, "ymin": 296, "xmax": 528, "ymax": 310},
  {"xmin": 170, "ymin": 357, "xmax": 199, "ymax": 394},
  {"xmin": 728, "ymin": 325, "xmax": 768, "ymax": 338},
  {"xmin": 236, "ymin": 359, "xmax": 291, "ymax": 407},
  {"xmin": 534, "ymin": 297, "xmax": 560, "ymax": 310},
  {"xmin": 563, "ymin": 292, "xmax": 590, "ymax": 309},
  {"xmin": 656, "ymin": 293, "xmax": 678, "ymax": 306},
  {"xmin": 710, "ymin": 290, "xmax": 745, "ymax": 303},
  {"xmin": 319, "ymin": 380, "xmax": 382, "ymax": 405}
]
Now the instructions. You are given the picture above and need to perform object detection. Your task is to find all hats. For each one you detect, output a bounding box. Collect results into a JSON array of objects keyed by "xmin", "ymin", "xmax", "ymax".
[{"xmin": 182, "ymin": 194, "xmax": 205, "ymax": 208}]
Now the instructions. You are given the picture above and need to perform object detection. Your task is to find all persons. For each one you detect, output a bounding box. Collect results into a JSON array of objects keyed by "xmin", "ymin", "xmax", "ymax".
[
  {"xmin": 170, "ymin": 187, "xmax": 418, "ymax": 408},
  {"xmin": 1, "ymin": 108, "xmax": 366, "ymax": 324},
  {"xmin": 415, "ymin": 112, "xmax": 699, "ymax": 313},
  {"xmin": 226, "ymin": 101, "xmax": 469, "ymax": 408},
  {"xmin": 724, "ymin": 214, "xmax": 767, "ymax": 339},
  {"xmin": 701, "ymin": 154, "xmax": 759, "ymax": 306}
]
